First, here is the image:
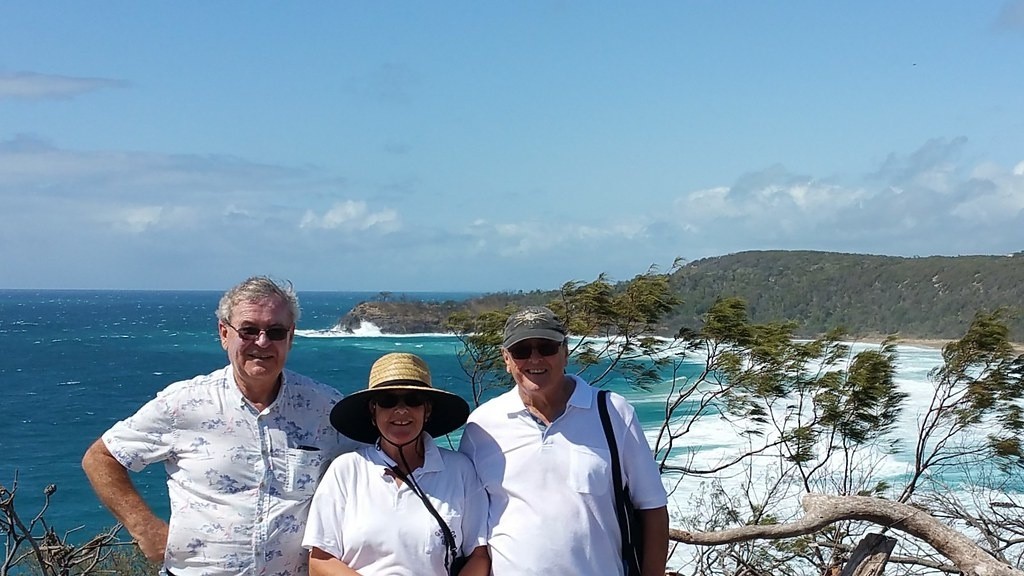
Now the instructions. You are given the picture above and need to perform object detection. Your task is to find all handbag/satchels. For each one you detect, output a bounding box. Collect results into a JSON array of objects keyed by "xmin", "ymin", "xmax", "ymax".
[
  {"xmin": 621, "ymin": 482, "xmax": 643, "ymax": 576},
  {"xmin": 450, "ymin": 556, "xmax": 468, "ymax": 576}
]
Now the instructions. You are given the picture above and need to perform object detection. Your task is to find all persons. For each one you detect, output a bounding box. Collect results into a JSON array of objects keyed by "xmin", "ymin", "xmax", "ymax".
[
  {"xmin": 457, "ymin": 306, "xmax": 669, "ymax": 576},
  {"xmin": 82, "ymin": 278, "xmax": 377, "ymax": 576},
  {"xmin": 302, "ymin": 353, "xmax": 492, "ymax": 576}
]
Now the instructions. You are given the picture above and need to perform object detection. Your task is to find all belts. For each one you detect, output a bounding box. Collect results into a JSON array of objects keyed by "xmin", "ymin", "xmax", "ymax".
[{"xmin": 166, "ymin": 567, "xmax": 175, "ymax": 576}]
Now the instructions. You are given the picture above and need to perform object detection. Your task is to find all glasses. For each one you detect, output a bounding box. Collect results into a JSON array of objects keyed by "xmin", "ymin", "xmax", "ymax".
[
  {"xmin": 372, "ymin": 392, "xmax": 426, "ymax": 409},
  {"xmin": 221, "ymin": 317, "xmax": 293, "ymax": 340},
  {"xmin": 508, "ymin": 339, "xmax": 565, "ymax": 359}
]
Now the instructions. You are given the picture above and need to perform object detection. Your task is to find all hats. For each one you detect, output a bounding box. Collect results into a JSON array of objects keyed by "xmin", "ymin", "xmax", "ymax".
[
  {"xmin": 329, "ymin": 352, "xmax": 470, "ymax": 444},
  {"xmin": 503, "ymin": 306, "xmax": 565, "ymax": 349}
]
